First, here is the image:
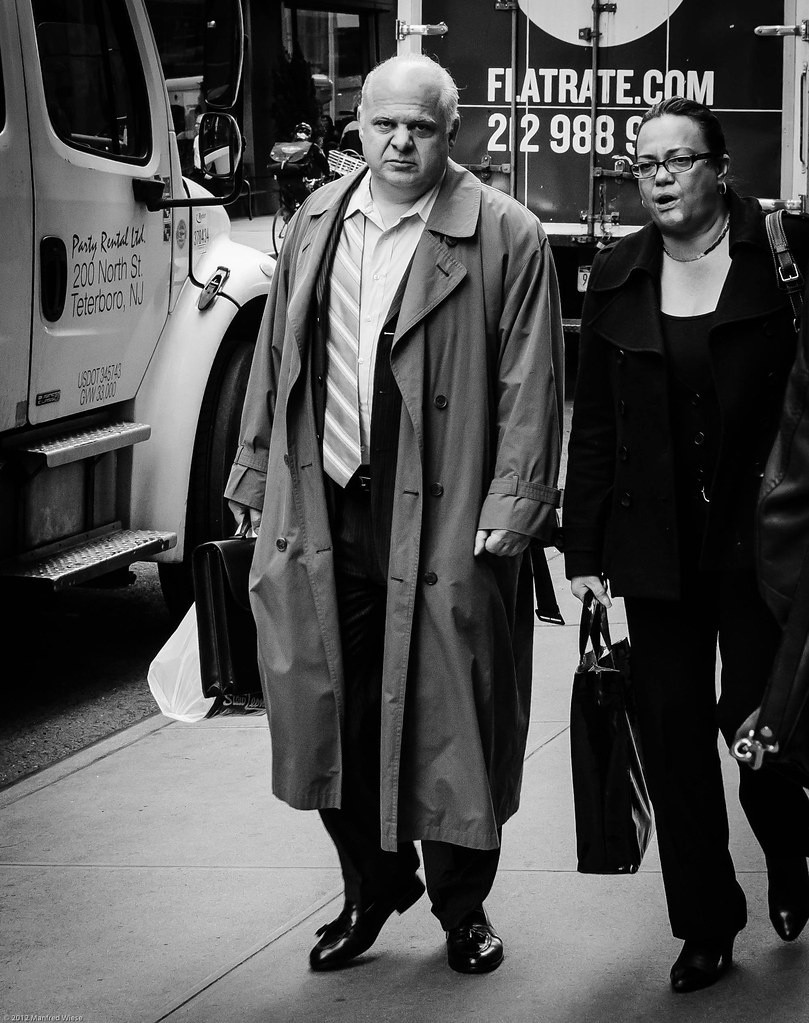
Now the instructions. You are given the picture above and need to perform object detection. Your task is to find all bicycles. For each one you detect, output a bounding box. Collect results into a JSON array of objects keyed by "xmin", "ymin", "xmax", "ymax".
[{"xmin": 265, "ymin": 122, "xmax": 372, "ymax": 264}]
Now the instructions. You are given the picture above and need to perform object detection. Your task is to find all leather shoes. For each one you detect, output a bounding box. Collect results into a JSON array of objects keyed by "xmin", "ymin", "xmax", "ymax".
[
  {"xmin": 445, "ymin": 905, "xmax": 503, "ymax": 974},
  {"xmin": 309, "ymin": 872, "xmax": 426, "ymax": 972}
]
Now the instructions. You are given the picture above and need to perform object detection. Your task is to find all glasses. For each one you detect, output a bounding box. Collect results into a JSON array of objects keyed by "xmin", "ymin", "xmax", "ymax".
[{"xmin": 630, "ymin": 152, "xmax": 716, "ymax": 179}]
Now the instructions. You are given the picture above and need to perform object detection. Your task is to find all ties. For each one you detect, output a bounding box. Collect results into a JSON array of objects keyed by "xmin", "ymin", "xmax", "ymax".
[{"xmin": 322, "ymin": 209, "xmax": 365, "ymax": 489}]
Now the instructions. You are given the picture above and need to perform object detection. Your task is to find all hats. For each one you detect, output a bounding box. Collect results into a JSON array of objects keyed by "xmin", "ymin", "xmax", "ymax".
[{"xmin": 293, "ymin": 123, "xmax": 312, "ymax": 139}]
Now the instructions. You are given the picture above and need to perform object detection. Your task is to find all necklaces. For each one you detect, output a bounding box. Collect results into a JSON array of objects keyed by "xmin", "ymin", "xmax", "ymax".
[{"xmin": 663, "ymin": 213, "xmax": 731, "ymax": 261}]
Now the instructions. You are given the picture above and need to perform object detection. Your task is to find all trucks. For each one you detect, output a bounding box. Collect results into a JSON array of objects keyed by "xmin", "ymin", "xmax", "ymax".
[
  {"xmin": 393, "ymin": 0, "xmax": 809, "ymax": 333},
  {"xmin": 1, "ymin": 0, "xmax": 283, "ymax": 624}
]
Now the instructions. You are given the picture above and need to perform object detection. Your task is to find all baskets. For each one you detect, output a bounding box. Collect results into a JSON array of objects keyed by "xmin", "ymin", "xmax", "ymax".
[{"xmin": 327, "ymin": 149, "xmax": 368, "ymax": 176}]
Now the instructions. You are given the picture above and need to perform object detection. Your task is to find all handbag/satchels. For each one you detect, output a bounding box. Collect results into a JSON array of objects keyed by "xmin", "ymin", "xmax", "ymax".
[
  {"xmin": 570, "ymin": 572, "xmax": 651, "ymax": 875},
  {"xmin": 191, "ymin": 509, "xmax": 263, "ymax": 698},
  {"xmin": 146, "ymin": 518, "xmax": 268, "ymax": 724}
]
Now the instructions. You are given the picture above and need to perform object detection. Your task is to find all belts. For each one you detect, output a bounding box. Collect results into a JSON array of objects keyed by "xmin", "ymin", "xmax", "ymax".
[{"xmin": 329, "ymin": 475, "xmax": 373, "ymax": 495}]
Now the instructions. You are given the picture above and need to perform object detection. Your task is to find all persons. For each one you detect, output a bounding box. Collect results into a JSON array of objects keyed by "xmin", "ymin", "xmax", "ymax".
[
  {"xmin": 564, "ymin": 98, "xmax": 809, "ymax": 990},
  {"xmin": 221, "ymin": 53, "xmax": 565, "ymax": 975}
]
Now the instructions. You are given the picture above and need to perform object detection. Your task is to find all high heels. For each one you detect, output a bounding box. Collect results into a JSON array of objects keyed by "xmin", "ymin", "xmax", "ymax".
[
  {"xmin": 766, "ymin": 842, "xmax": 809, "ymax": 942},
  {"xmin": 669, "ymin": 896, "xmax": 747, "ymax": 996}
]
